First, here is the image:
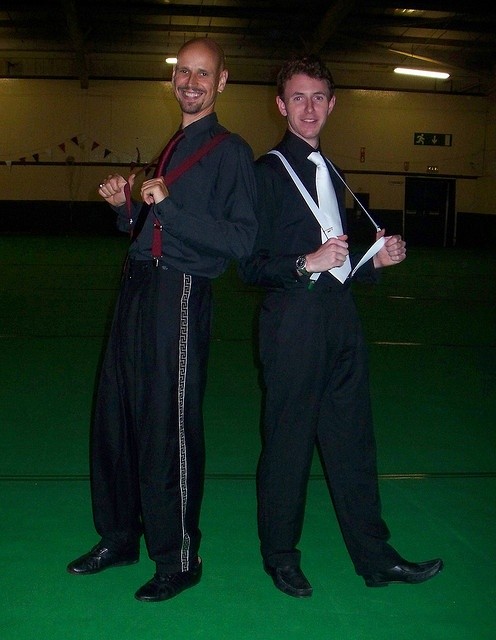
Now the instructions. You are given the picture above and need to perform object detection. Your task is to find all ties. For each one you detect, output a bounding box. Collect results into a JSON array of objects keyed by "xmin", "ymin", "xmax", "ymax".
[
  {"xmin": 307, "ymin": 151, "xmax": 352, "ymax": 285},
  {"xmin": 129, "ymin": 130, "xmax": 185, "ymax": 248}
]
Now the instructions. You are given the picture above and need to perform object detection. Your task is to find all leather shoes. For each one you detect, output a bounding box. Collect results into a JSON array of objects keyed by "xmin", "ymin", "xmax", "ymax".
[
  {"xmin": 135, "ymin": 556, "xmax": 202, "ymax": 601},
  {"xmin": 67, "ymin": 540, "xmax": 139, "ymax": 574},
  {"xmin": 263, "ymin": 559, "xmax": 313, "ymax": 598},
  {"xmin": 364, "ymin": 554, "xmax": 443, "ymax": 587}
]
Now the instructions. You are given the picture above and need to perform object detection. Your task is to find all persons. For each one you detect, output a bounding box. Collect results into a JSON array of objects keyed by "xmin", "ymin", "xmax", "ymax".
[
  {"xmin": 66, "ymin": 37, "xmax": 260, "ymax": 604},
  {"xmin": 237, "ymin": 53, "xmax": 444, "ymax": 598}
]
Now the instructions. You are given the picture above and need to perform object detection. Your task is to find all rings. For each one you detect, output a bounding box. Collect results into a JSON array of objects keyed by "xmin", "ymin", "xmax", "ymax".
[{"xmin": 98, "ymin": 182, "xmax": 107, "ymax": 188}]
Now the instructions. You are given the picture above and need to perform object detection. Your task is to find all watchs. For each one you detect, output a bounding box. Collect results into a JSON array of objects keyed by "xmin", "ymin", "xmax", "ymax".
[{"xmin": 295, "ymin": 254, "xmax": 309, "ymax": 275}]
